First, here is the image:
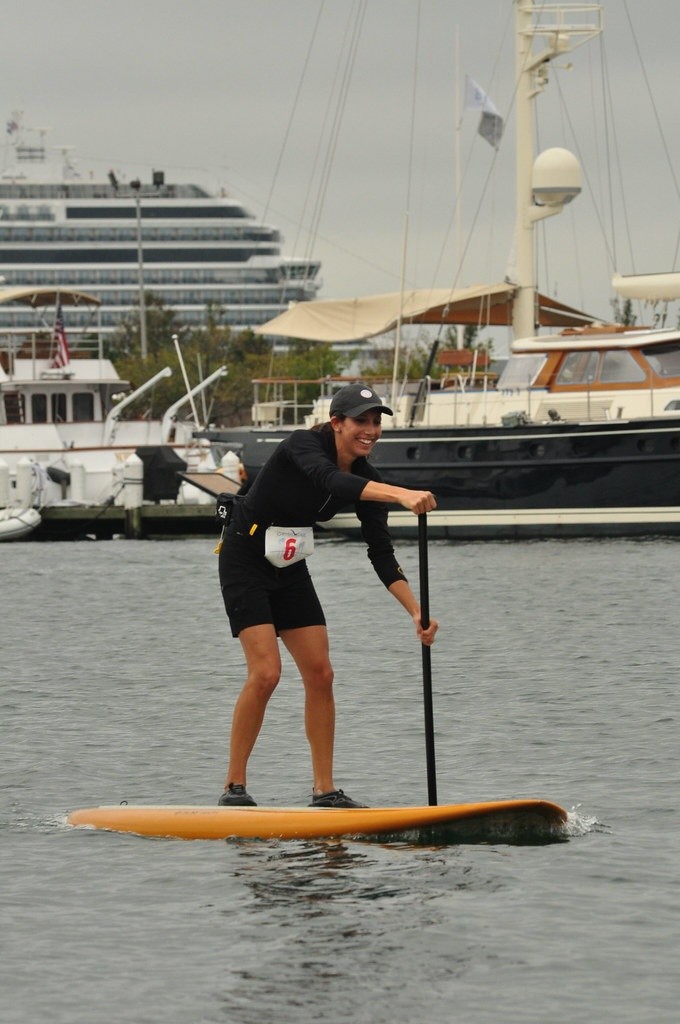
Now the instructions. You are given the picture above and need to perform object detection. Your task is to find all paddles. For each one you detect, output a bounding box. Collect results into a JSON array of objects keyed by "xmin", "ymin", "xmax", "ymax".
[{"xmin": 413, "ymin": 508, "xmax": 442, "ymax": 804}]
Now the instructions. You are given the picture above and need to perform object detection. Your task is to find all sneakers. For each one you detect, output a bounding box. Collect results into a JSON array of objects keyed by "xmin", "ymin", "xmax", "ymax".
[
  {"xmin": 219, "ymin": 784, "xmax": 259, "ymax": 806},
  {"xmin": 311, "ymin": 789, "xmax": 369, "ymax": 809}
]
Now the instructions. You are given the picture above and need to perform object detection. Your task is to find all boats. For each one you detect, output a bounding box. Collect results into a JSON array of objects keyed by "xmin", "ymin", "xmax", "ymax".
[
  {"xmin": 73, "ymin": 801, "xmax": 569, "ymax": 840},
  {"xmin": 0, "ymin": 280, "xmax": 246, "ymax": 544},
  {"xmin": 192, "ymin": 2, "xmax": 680, "ymax": 536}
]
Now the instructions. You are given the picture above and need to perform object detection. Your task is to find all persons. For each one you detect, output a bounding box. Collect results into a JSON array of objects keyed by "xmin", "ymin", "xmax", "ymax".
[{"xmin": 217, "ymin": 385, "xmax": 440, "ymax": 809}]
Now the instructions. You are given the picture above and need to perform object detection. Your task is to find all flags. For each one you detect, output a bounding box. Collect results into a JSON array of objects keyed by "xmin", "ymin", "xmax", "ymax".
[{"xmin": 50, "ymin": 299, "xmax": 70, "ymax": 369}]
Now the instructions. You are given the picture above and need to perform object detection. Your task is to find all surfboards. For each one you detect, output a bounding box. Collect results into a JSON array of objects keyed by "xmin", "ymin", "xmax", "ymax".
[{"xmin": 68, "ymin": 795, "xmax": 570, "ymax": 846}]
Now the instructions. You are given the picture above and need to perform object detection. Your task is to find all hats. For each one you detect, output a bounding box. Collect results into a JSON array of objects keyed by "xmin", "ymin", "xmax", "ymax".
[{"xmin": 330, "ymin": 385, "xmax": 393, "ymax": 418}]
